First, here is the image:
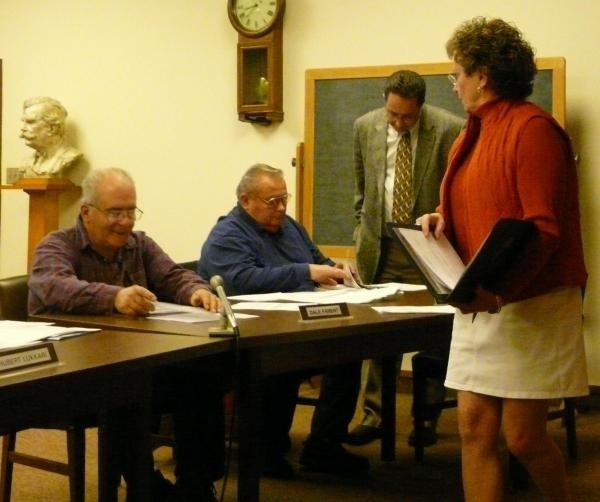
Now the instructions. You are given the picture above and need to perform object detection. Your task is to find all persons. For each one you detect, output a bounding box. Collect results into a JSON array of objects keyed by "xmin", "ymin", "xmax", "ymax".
[
  {"xmin": 414, "ymin": 16, "xmax": 590, "ymax": 502},
  {"xmin": 20, "ymin": 99, "xmax": 82, "ymax": 180},
  {"xmin": 198, "ymin": 163, "xmax": 372, "ymax": 477},
  {"xmin": 345, "ymin": 69, "xmax": 470, "ymax": 447},
  {"xmin": 28, "ymin": 168, "xmax": 223, "ymax": 502}
]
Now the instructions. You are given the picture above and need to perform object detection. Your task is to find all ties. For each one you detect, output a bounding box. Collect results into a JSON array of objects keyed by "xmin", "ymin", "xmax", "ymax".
[{"xmin": 391, "ymin": 130, "xmax": 417, "ymax": 228}]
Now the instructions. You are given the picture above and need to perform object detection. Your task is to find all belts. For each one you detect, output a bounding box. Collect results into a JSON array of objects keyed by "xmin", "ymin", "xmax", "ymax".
[{"xmin": 383, "ymin": 221, "xmax": 433, "ymax": 233}]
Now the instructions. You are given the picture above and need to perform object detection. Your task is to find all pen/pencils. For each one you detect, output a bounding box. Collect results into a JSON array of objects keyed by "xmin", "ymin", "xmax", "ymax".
[{"xmin": 126, "ymin": 270, "xmax": 138, "ymax": 284}]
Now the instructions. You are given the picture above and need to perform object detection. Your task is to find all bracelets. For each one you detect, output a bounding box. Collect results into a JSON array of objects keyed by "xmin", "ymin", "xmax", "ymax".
[{"xmin": 489, "ymin": 295, "xmax": 503, "ymax": 315}]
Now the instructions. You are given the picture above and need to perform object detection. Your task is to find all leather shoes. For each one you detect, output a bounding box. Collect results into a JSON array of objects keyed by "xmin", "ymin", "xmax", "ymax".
[
  {"xmin": 407, "ymin": 418, "xmax": 440, "ymax": 447},
  {"xmin": 344, "ymin": 421, "xmax": 383, "ymax": 447}
]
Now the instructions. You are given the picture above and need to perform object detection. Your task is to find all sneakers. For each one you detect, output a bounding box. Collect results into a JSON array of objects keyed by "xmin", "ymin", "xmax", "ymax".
[
  {"xmin": 255, "ymin": 447, "xmax": 296, "ymax": 481},
  {"xmin": 123, "ymin": 468, "xmax": 188, "ymax": 502},
  {"xmin": 171, "ymin": 464, "xmax": 221, "ymax": 502},
  {"xmin": 298, "ymin": 437, "xmax": 368, "ymax": 472}
]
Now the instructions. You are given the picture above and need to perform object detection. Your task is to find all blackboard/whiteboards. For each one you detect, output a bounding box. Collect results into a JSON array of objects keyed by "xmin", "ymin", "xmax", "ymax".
[{"xmin": 302, "ymin": 56, "xmax": 567, "ymax": 260}]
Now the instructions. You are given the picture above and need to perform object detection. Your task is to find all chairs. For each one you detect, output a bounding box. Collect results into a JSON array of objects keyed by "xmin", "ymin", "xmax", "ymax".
[
  {"xmin": 0, "ymin": 274, "xmax": 123, "ymax": 502},
  {"xmin": 410, "ymin": 349, "xmax": 578, "ymax": 468}
]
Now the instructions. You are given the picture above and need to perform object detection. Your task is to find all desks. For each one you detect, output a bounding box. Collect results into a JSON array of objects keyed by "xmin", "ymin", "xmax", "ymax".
[
  {"xmin": 28, "ymin": 279, "xmax": 581, "ymax": 502},
  {"xmin": 0, "ymin": 317, "xmax": 236, "ymax": 502}
]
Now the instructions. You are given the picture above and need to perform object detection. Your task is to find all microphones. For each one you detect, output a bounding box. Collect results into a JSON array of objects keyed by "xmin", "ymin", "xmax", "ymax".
[{"xmin": 210, "ymin": 274, "xmax": 240, "ymax": 336}]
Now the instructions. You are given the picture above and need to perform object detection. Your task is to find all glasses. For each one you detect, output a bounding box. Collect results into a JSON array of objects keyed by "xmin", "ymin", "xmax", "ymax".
[
  {"xmin": 249, "ymin": 191, "xmax": 293, "ymax": 208},
  {"xmin": 446, "ymin": 69, "xmax": 485, "ymax": 86},
  {"xmin": 86, "ymin": 202, "xmax": 146, "ymax": 222}
]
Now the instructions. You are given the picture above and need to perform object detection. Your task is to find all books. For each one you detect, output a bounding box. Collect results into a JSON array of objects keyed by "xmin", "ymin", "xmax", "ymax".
[{"xmin": 385, "ymin": 217, "xmax": 539, "ymax": 308}]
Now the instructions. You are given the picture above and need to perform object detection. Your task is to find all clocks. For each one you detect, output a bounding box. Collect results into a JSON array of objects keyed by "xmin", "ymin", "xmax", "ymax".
[{"xmin": 228, "ymin": 0, "xmax": 284, "ymax": 126}]
